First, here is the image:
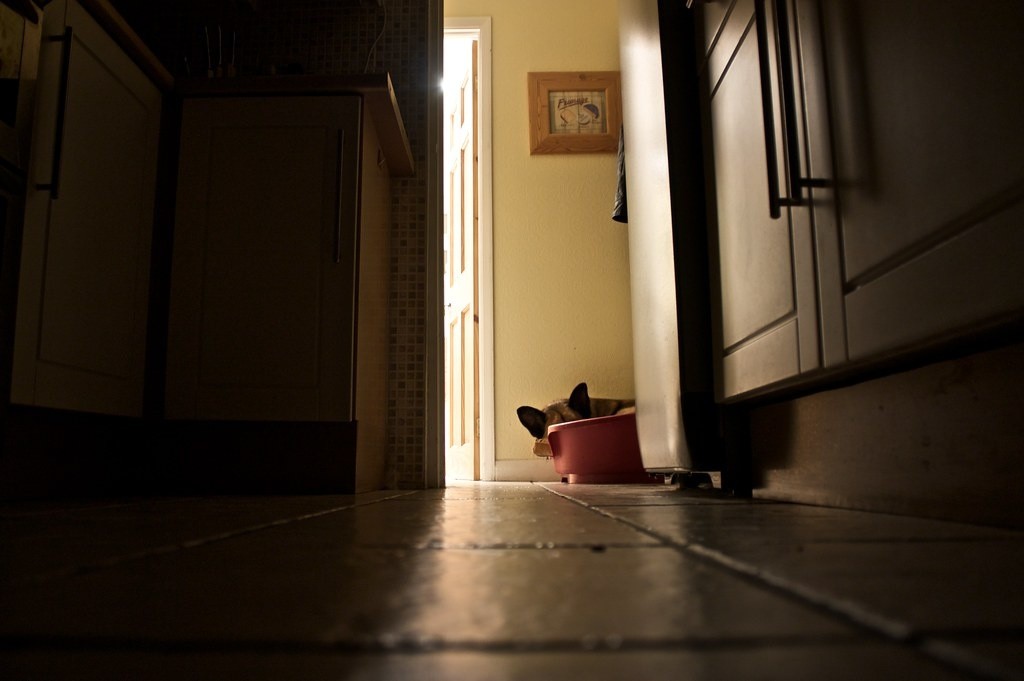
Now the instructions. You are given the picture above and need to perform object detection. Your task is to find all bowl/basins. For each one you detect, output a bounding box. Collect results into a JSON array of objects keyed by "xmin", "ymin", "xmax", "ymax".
[{"xmin": 548, "ymin": 412, "xmax": 643, "ymax": 485}]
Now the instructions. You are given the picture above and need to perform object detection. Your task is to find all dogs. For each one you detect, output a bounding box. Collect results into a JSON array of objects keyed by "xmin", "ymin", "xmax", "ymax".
[{"xmin": 517, "ymin": 382, "xmax": 635, "ymax": 457}]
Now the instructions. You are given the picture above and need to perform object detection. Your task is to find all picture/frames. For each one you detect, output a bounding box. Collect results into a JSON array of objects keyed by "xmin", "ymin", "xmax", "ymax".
[{"xmin": 526, "ymin": 70, "xmax": 622, "ymax": 157}]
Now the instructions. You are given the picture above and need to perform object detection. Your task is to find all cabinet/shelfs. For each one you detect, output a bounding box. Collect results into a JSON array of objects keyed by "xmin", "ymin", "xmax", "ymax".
[
  {"xmin": 686, "ymin": 0, "xmax": 1024, "ymax": 527},
  {"xmin": 0, "ymin": 0, "xmax": 165, "ymax": 495},
  {"xmin": 169, "ymin": 93, "xmax": 393, "ymax": 497}
]
